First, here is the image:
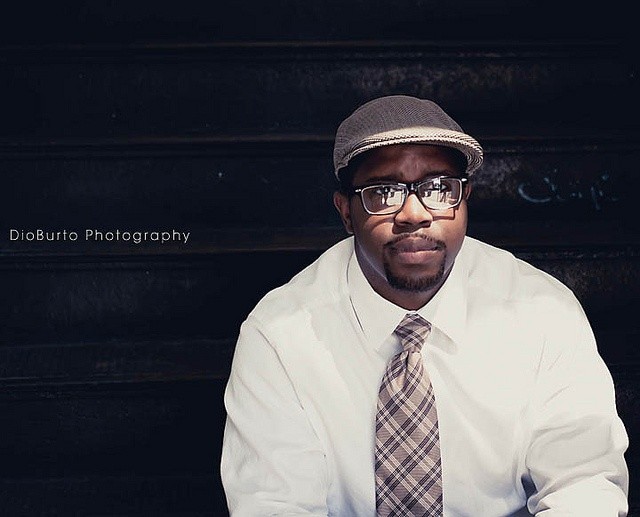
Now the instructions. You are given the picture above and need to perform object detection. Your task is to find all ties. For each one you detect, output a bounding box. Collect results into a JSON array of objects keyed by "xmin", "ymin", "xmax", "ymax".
[{"xmin": 373, "ymin": 315, "xmax": 443, "ymax": 516}]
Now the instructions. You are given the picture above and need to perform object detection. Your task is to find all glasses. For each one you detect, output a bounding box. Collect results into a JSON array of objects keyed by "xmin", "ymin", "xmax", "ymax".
[{"xmin": 341, "ymin": 174, "xmax": 470, "ymax": 216}]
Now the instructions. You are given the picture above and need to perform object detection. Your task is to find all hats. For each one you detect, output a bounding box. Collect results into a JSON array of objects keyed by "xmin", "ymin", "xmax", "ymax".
[{"xmin": 332, "ymin": 96, "xmax": 483, "ymax": 180}]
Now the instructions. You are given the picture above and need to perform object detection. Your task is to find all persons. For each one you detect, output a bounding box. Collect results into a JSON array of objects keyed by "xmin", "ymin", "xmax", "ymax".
[{"xmin": 219, "ymin": 94, "xmax": 629, "ymax": 517}]
